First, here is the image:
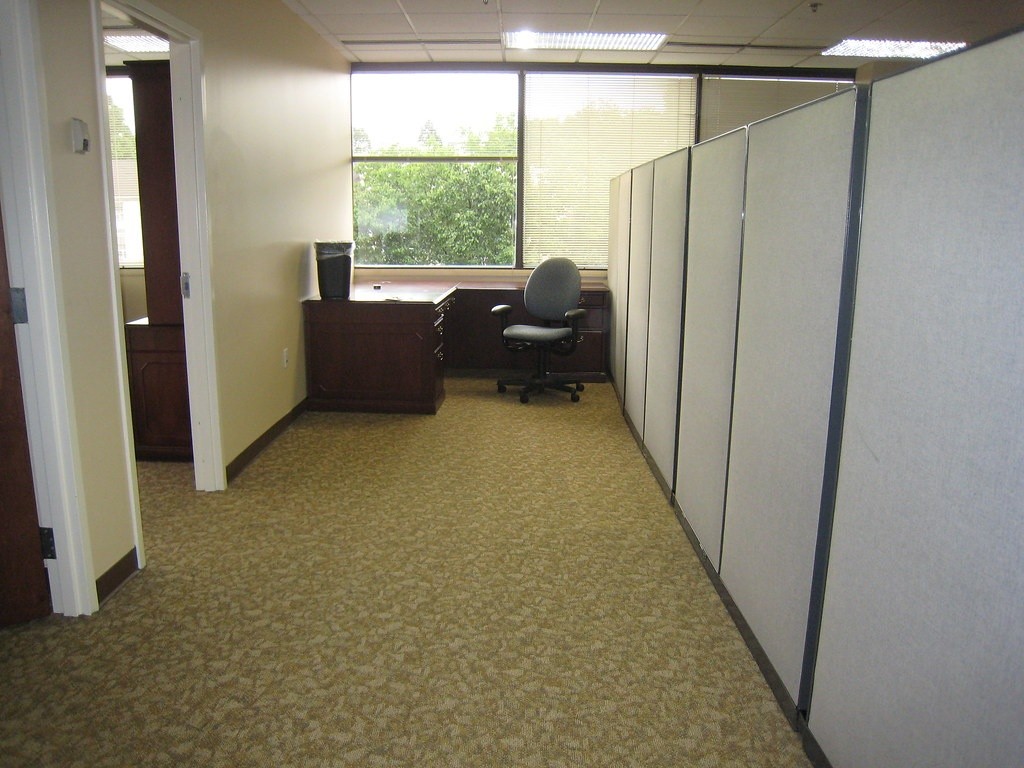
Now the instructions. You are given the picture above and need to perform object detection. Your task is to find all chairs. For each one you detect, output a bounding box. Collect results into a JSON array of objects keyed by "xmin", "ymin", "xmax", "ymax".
[{"xmin": 490, "ymin": 257, "xmax": 585, "ymax": 402}]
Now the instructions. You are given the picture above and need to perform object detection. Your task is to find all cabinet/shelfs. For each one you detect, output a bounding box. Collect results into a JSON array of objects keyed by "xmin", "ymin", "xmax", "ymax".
[
  {"xmin": 456, "ymin": 281, "xmax": 609, "ymax": 383},
  {"xmin": 301, "ymin": 280, "xmax": 461, "ymax": 414},
  {"xmin": 125, "ymin": 315, "xmax": 194, "ymax": 463},
  {"xmin": 105, "ymin": 59, "xmax": 192, "ymax": 324}
]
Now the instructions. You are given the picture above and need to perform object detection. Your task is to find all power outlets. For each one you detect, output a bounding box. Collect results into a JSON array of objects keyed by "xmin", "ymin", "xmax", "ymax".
[{"xmin": 283, "ymin": 348, "xmax": 288, "ymax": 367}]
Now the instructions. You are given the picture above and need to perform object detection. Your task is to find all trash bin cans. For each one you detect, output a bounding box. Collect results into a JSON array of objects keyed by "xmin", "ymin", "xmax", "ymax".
[{"xmin": 314, "ymin": 242, "xmax": 353, "ymax": 300}]
